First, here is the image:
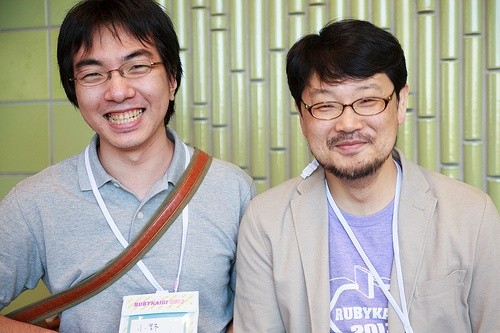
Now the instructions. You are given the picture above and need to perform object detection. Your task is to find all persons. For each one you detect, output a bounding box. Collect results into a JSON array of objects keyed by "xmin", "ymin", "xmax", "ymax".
[
  {"xmin": 233, "ymin": 20, "xmax": 500, "ymax": 333},
  {"xmin": 0, "ymin": 0, "xmax": 258, "ymax": 333}
]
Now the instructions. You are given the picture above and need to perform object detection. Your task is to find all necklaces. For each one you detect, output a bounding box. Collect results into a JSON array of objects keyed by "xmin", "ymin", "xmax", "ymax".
[{"xmin": 324, "ymin": 158, "xmax": 418, "ymax": 333}]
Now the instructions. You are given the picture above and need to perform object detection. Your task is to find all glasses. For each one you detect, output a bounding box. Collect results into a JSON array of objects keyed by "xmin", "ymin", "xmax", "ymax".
[
  {"xmin": 301, "ymin": 87, "xmax": 395, "ymax": 121},
  {"xmin": 68, "ymin": 59, "xmax": 164, "ymax": 87}
]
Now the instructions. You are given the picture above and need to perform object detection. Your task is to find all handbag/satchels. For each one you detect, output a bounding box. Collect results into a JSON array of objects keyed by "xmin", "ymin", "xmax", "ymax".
[{"xmin": 34, "ymin": 316, "xmax": 60, "ymax": 330}]
